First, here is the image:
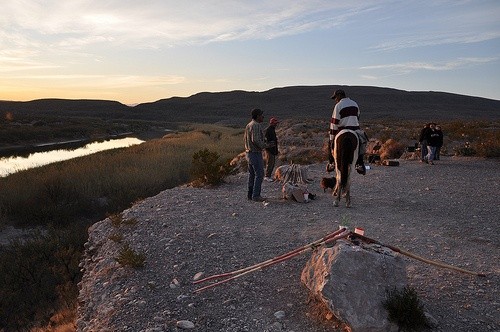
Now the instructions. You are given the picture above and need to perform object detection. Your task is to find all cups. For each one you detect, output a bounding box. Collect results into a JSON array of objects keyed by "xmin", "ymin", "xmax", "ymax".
[{"xmin": 304, "ymin": 194, "xmax": 308, "ymax": 200}]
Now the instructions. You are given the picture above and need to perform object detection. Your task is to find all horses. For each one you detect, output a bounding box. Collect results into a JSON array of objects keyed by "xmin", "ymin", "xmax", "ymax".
[{"xmin": 330, "ymin": 128, "xmax": 360, "ymax": 209}]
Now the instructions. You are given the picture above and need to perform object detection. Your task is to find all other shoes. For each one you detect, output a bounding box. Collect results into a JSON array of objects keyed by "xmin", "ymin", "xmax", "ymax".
[
  {"xmin": 264, "ymin": 177, "xmax": 273, "ymax": 182},
  {"xmin": 252, "ymin": 197, "xmax": 264, "ymax": 202}
]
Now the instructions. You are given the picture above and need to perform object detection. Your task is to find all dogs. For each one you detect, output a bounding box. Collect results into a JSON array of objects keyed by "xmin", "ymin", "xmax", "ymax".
[{"xmin": 320, "ymin": 176, "xmax": 337, "ymax": 192}]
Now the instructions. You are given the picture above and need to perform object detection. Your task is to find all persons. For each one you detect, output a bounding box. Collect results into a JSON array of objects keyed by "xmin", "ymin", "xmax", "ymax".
[
  {"xmin": 327, "ymin": 89, "xmax": 366, "ymax": 175},
  {"xmin": 265, "ymin": 118, "xmax": 279, "ymax": 182},
  {"xmin": 419, "ymin": 122, "xmax": 443, "ymax": 164},
  {"xmin": 373, "ymin": 141, "xmax": 381, "ymax": 154},
  {"xmin": 244, "ymin": 108, "xmax": 273, "ymax": 202}
]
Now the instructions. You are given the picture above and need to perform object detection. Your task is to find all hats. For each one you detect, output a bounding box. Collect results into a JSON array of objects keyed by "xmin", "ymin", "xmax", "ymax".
[
  {"xmin": 330, "ymin": 89, "xmax": 345, "ymax": 99},
  {"xmin": 270, "ymin": 118, "xmax": 280, "ymax": 124}
]
